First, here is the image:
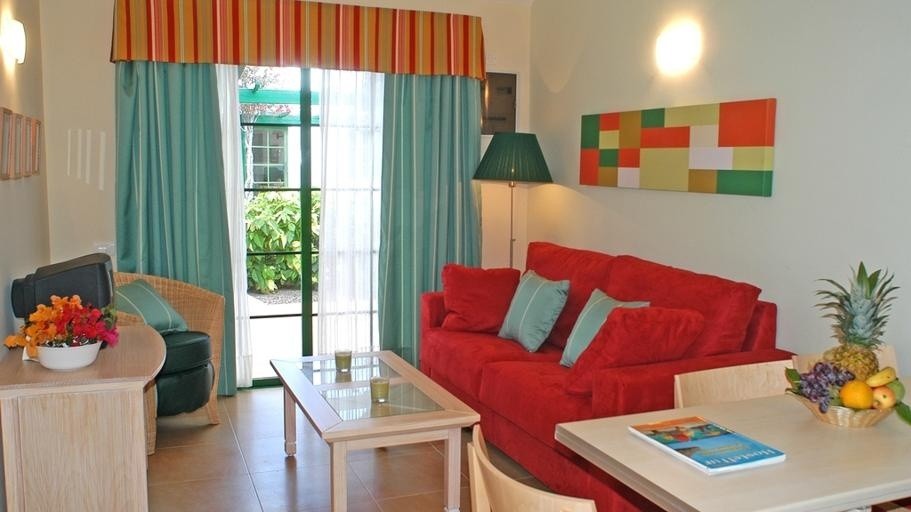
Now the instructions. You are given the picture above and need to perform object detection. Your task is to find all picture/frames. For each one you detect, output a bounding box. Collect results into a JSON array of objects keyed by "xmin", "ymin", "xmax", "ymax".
[{"xmin": 0, "ymin": 107, "xmax": 42, "ymax": 181}]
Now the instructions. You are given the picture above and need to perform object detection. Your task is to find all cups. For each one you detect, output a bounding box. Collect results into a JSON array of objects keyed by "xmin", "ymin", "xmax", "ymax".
[
  {"xmin": 335, "ymin": 350, "xmax": 352, "ymax": 372},
  {"xmin": 371, "ymin": 377, "xmax": 390, "ymax": 403}
]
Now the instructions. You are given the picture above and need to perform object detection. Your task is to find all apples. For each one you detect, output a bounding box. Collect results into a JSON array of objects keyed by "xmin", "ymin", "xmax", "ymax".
[
  {"xmin": 886, "ymin": 378, "xmax": 904, "ymax": 402},
  {"xmin": 872, "ymin": 386, "xmax": 895, "ymax": 409}
]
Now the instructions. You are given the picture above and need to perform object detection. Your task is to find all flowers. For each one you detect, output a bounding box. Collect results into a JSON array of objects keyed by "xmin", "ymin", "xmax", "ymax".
[{"xmin": 4, "ymin": 293, "xmax": 119, "ymax": 358}]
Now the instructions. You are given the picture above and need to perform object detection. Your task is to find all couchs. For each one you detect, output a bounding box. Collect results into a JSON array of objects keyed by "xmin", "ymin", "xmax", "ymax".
[{"xmin": 416, "ymin": 241, "xmax": 797, "ymax": 512}]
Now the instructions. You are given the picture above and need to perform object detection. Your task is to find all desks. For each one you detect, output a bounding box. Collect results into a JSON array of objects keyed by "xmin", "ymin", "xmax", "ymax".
[{"xmin": 0, "ymin": 323, "xmax": 170, "ymax": 512}]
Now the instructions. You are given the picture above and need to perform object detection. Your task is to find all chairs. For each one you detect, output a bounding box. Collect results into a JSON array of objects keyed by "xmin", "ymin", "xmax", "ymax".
[
  {"xmin": 466, "ymin": 424, "xmax": 598, "ymax": 512},
  {"xmin": 673, "ymin": 359, "xmax": 793, "ymax": 409},
  {"xmin": 109, "ymin": 266, "xmax": 227, "ymax": 426},
  {"xmin": 792, "ymin": 345, "xmax": 898, "ymax": 377}
]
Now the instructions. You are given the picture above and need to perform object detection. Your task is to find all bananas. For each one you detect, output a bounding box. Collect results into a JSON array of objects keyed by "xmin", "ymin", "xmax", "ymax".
[{"xmin": 865, "ymin": 367, "xmax": 896, "ymax": 387}]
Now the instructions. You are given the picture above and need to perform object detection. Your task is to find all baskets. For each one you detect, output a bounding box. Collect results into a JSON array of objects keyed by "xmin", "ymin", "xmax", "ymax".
[{"xmin": 785, "ymin": 388, "xmax": 896, "ymax": 428}]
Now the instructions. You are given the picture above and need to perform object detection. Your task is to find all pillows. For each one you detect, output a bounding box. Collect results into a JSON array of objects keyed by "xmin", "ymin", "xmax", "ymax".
[
  {"xmin": 496, "ymin": 269, "xmax": 571, "ymax": 353},
  {"xmin": 561, "ymin": 306, "xmax": 706, "ymax": 394},
  {"xmin": 558, "ymin": 287, "xmax": 651, "ymax": 369},
  {"xmin": 440, "ymin": 263, "xmax": 520, "ymax": 333},
  {"xmin": 113, "ymin": 278, "xmax": 189, "ymax": 335}
]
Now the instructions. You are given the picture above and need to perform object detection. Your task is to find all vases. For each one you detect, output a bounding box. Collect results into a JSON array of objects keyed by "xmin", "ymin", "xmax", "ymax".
[{"xmin": 34, "ymin": 339, "xmax": 103, "ymax": 371}]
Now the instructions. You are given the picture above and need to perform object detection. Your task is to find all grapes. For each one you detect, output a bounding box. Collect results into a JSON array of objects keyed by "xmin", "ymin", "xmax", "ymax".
[{"xmin": 790, "ymin": 362, "xmax": 840, "ymax": 414}]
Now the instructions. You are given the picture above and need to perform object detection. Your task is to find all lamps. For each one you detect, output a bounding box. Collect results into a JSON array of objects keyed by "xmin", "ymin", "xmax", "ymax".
[{"xmin": 471, "ymin": 130, "xmax": 554, "ymax": 268}]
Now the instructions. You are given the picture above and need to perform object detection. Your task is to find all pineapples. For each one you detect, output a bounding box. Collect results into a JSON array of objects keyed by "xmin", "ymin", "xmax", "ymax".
[{"xmin": 811, "ymin": 261, "xmax": 880, "ymax": 380}]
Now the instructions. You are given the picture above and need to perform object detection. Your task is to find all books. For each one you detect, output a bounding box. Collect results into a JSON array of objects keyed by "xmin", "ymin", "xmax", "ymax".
[{"xmin": 627, "ymin": 415, "xmax": 785, "ymax": 475}]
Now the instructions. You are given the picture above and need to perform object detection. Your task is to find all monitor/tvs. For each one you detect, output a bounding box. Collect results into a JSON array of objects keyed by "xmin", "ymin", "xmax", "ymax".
[{"xmin": 11, "ymin": 253, "xmax": 117, "ymax": 320}]
